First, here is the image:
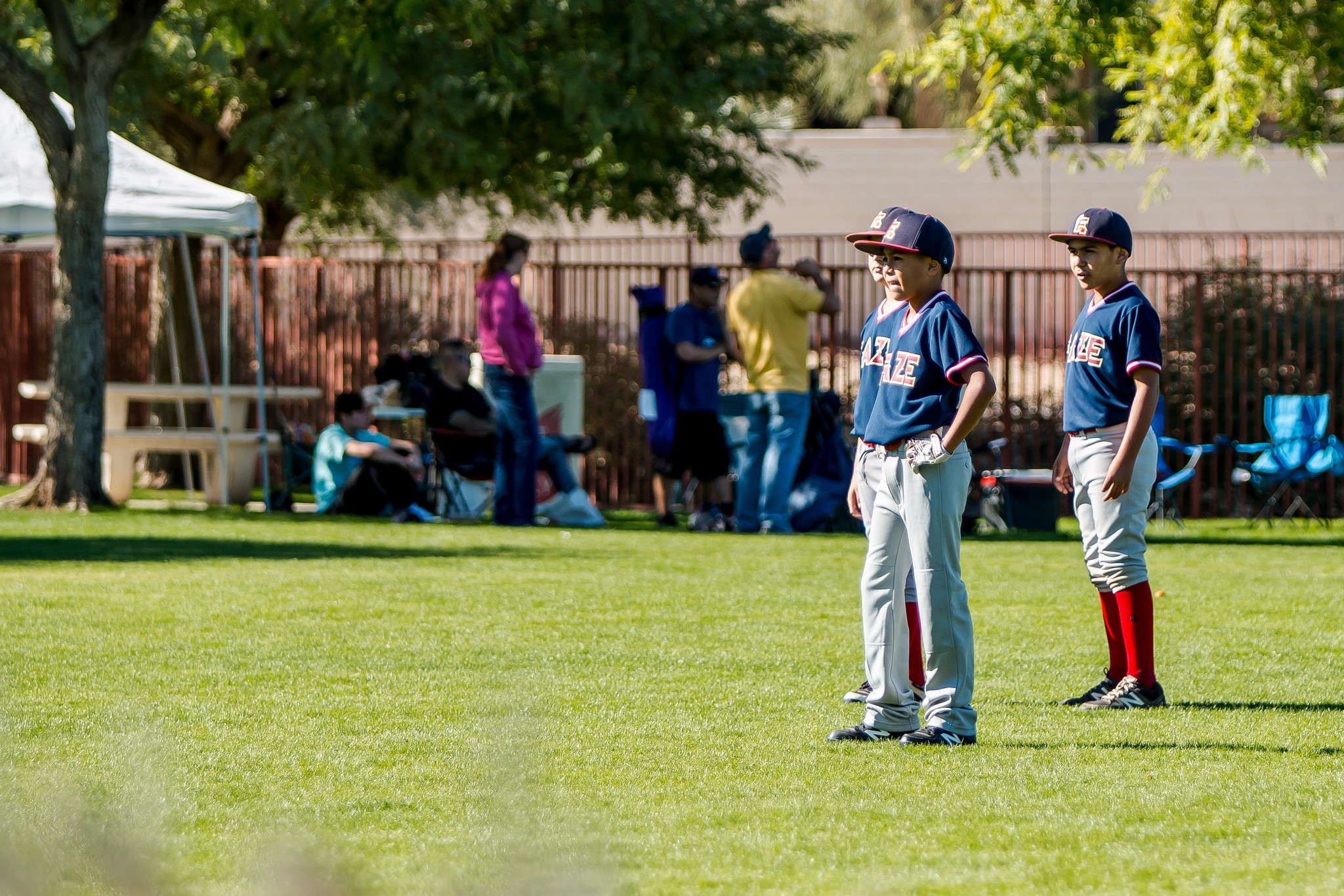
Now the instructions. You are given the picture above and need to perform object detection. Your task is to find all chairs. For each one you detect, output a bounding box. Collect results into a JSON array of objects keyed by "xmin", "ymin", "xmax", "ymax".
[
  {"xmin": 1233, "ymin": 395, "xmax": 1344, "ymax": 528},
  {"xmin": 372, "ymin": 354, "xmax": 495, "ymax": 522},
  {"xmin": 1149, "ymin": 394, "xmax": 1213, "ymax": 520}
]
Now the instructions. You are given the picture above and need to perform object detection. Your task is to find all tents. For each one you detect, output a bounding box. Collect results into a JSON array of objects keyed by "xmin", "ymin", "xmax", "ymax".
[{"xmin": 0, "ymin": 89, "xmax": 270, "ymax": 513}]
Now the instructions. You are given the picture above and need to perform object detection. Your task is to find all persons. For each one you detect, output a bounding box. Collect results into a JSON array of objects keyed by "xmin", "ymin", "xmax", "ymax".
[
  {"xmin": 829, "ymin": 212, "xmax": 996, "ymax": 745},
  {"xmin": 633, "ymin": 284, "xmax": 687, "ymax": 524},
  {"xmin": 724, "ymin": 222, "xmax": 841, "ymax": 534},
  {"xmin": 475, "ymin": 231, "xmax": 542, "ymax": 527},
  {"xmin": 666, "ymin": 266, "xmax": 733, "ymax": 532},
  {"xmin": 312, "ymin": 390, "xmax": 439, "ymax": 522},
  {"xmin": 424, "ymin": 336, "xmax": 604, "ymax": 495},
  {"xmin": 842, "ymin": 206, "xmax": 925, "ymax": 702},
  {"xmin": 1049, "ymin": 207, "xmax": 1165, "ymax": 710}
]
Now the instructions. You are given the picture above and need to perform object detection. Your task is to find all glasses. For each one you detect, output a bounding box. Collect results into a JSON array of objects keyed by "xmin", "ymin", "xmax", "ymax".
[{"xmin": 455, "ymin": 354, "xmax": 472, "ymax": 366}]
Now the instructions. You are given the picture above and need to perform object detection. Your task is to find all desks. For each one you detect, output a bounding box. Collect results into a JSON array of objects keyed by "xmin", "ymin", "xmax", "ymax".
[{"xmin": 17, "ymin": 381, "xmax": 327, "ymax": 501}]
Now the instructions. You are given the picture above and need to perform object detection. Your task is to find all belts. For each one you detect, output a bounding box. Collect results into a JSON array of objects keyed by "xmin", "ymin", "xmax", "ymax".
[
  {"xmin": 1068, "ymin": 429, "xmax": 1097, "ymax": 436},
  {"xmin": 863, "ymin": 439, "xmax": 901, "ymax": 451}
]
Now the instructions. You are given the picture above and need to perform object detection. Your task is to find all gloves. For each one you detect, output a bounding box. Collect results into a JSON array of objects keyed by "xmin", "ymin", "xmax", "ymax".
[{"xmin": 904, "ymin": 433, "xmax": 954, "ymax": 474}]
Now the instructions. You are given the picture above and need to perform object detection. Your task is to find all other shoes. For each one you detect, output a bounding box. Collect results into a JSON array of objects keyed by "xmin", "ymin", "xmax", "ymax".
[{"xmin": 659, "ymin": 499, "xmax": 784, "ymax": 536}]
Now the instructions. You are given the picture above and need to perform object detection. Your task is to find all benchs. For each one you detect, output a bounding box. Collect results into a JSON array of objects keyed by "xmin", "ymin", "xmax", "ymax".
[{"xmin": 10, "ymin": 421, "xmax": 290, "ymax": 452}]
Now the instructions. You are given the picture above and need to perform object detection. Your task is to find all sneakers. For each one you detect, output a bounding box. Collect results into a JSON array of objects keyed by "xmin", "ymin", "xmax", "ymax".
[
  {"xmin": 844, "ymin": 680, "xmax": 873, "ymax": 703},
  {"xmin": 561, "ymin": 435, "xmax": 596, "ymax": 454},
  {"xmin": 828, "ymin": 722, "xmax": 915, "ymax": 744},
  {"xmin": 1080, "ymin": 675, "xmax": 1166, "ymax": 709},
  {"xmin": 900, "ymin": 725, "xmax": 976, "ymax": 747},
  {"xmin": 1058, "ymin": 668, "xmax": 1121, "ymax": 707},
  {"xmin": 909, "ymin": 680, "xmax": 927, "ymax": 702}
]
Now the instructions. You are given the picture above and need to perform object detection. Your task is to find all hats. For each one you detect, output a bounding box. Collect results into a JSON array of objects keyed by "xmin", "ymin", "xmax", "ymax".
[
  {"xmin": 853, "ymin": 213, "xmax": 955, "ymax": 274},
  {"xmin": 1047, "ymin": 207, "xmax": 1132, "ymax": 258},
  {"xmin": 740, "ymin": 223, "xmax": 773, "ymax": 266},
  {"xmin": 692, "ymin": 267, "xmax": 729, "ymax": 287},
  {"xmin": 845, "ymin": 207, "xmax": 916, "ymax": 243}
]
